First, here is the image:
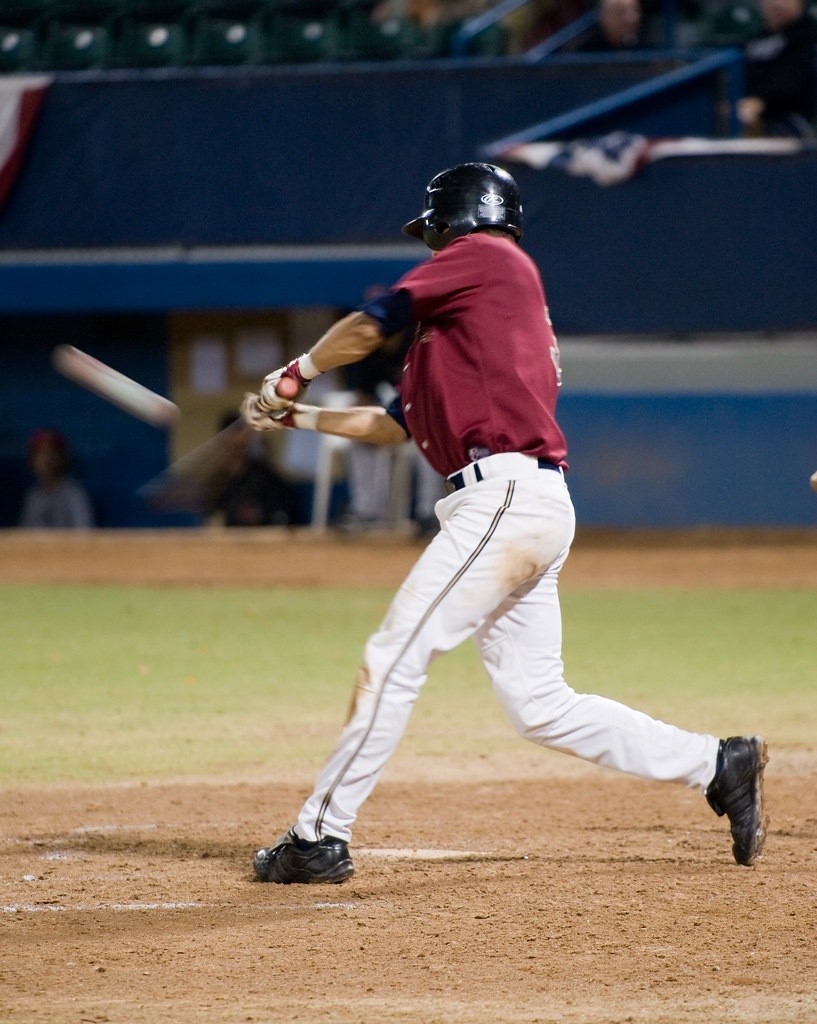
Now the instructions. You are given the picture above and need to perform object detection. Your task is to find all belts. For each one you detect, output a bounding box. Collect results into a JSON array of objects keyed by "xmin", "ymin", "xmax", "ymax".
[{"xmin": 443, "ymin": 457, "xmax": 561, "ymax": 497}]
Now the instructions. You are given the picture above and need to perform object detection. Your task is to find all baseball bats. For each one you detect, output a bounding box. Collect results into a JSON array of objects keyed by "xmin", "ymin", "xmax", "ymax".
[{"xmin": 140, "ymin": 376, "xmax": 303, "ymax": 516}]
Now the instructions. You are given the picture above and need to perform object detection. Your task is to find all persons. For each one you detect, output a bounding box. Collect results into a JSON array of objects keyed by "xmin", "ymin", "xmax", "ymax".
[
  {"xmin": 739, "ymin": 0, "xmax": 817, "ymax": 138},
  {"xmin": 252, "ymin": 161, "xmax": 773, "ymax": 883},
  {"xmin": 372, "ymin": 0, "xmax": 560, "ymax": 56},
  {"xmin": 18, "ymin": 426, "xmax": 90, "ymax": 525},
  {"xmin": 207, "ymin": 409, "xmax": 300, "ymax": 528},
  {"xmin": 577, "ymin": 0, "xmax": 665, "ymax": 54}
]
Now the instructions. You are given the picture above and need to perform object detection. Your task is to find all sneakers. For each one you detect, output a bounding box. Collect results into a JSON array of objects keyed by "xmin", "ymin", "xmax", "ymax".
[
  {"xmin": 705, "ymin": 734, "xmax": 771, "ymax": 866},
  {"xmin": 252, "ymin": 825, "xmax": 354, "ymax": 885}
]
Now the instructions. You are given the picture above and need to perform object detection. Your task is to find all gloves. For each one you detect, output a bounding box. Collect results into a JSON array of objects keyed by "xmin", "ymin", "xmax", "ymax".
[{"xmin": 239, "ymin": 352, "xmax": 325, "ymax": 432}]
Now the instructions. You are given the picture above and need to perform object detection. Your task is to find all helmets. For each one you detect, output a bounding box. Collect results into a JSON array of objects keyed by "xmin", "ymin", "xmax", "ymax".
[{"xmin": 400, "ymin": 162, "xmax": 523, "ymax": 251}]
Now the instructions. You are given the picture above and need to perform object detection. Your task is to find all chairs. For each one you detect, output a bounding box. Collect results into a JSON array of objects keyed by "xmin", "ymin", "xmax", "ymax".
[{"xmin": 0, "ymin": 14, "xmax": 511, "ymax": 77}]
